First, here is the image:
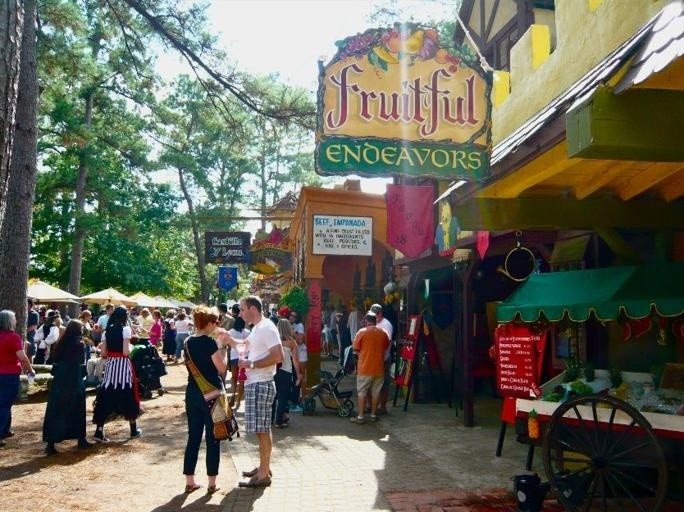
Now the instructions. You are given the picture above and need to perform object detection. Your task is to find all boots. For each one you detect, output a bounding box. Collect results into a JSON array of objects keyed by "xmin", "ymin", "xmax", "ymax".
[
  {"xmin": 130, "ymin": 422, "xmax": 142, "ymax": 438},
  {"xmin": 93, "ymin": 425, "xmax": 110, "ymax": 443}
]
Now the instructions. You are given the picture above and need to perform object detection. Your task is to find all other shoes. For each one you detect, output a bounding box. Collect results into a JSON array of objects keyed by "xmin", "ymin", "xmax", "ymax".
[
  {"xmin": 243, "ymin": 468, "xmax": 272, "ymax": 477},
  {"xmin": 276, "ymin": 421, "xmax": 288, "ymax": 428},
  {"xmin": 46, "ymin": 445, "xmax": 59, "ymax": 454},
  {"xmin": 239, "ymin": 474, "xmax": 271, "ymax": 486},
  {"xmin": 77, "ymin": 439, "xmax": 95, "ymax": 448},
  {"xmin": 350, "ymin": 416, "xmax": 364, "ymax": 423},
  {"xmin": 186, "ymin": 484, "xmax": 200, "ymax": 492},
  {"xmin": 207, "ymin": 485, "xmax": 218, "ymax": 491},
  {"xmin": 370, "ymin": 416, "xmax": 378, "ymax": 422}
]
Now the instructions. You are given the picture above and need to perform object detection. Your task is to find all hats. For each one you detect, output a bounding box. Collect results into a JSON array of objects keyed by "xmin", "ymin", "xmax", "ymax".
[{"xmin": 365, "ymin": 304, "xmax": 382, "ymax": 321}]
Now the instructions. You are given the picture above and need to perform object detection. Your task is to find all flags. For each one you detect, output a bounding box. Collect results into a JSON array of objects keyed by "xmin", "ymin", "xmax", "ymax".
[
  {"xmin": 477, "ymin": 231, "xmax": 490, "ymax": 260},
  {"xmin": 387, "ymin": 185, "xmax": 435, "ymax": 258},
  {"xmin": 219, "ymin": 267, "xmax": 237, "ymax": 292}
]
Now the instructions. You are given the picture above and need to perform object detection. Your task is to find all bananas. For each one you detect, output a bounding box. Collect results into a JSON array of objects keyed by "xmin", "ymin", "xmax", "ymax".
[{"xmin": 373, "ymin": 44, "xmax": 399, "ymax": 64}]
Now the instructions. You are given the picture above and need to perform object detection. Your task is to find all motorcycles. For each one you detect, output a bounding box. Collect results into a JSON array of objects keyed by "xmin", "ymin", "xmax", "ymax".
[{"xmin": 82, "ymin": 349, "xmax": 106, "ymax": 388}]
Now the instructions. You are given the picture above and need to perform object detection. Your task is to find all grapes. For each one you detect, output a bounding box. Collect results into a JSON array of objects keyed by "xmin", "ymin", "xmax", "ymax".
[
  {"xmin": 438, "ymin": 30, "xmax": 478, "ymax": 68},
  {"xmin": 339, "ymin": 28, "xmax": 389, "ymax": 60}
]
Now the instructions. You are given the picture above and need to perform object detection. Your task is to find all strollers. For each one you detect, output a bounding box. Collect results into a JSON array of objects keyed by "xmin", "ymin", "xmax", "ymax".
[
  {"xmin": 301, "ymin": 345, "xmax": 357, "ymax": 417},
  {"xmin": 130, "ymin": 339, "xmax": 167, "ymax": 400}
]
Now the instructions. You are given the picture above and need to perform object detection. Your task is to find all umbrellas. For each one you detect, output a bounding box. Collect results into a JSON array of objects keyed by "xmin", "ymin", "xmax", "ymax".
[{"xmin": 27, "ymin": 276, "xmax": 198, "ymax": 309}]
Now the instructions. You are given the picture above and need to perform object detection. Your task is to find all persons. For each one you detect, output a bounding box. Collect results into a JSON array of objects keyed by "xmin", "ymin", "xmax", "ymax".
[
  {"xmin": 181, "ymin": 307, "xmax": 230, "ymax": 495},
  {"xmin": 0, "ymin": 311, "xmax": 36, "ymax": 446},
  {"xmin": 92, "ymin": 306, "xmax": 144, "ymax": 443},
  {"xmin": 264, "ymin": 307, "xmax": 308, "ymax": 411},
  {"xmin": 42, "ymin": 319, "xmax": 95, "ymax": 455},
  {"xmin": 218, "ymin": 303, "xmax": 251, "ymax": 408},
  {"xmin": 26, "ymin": 299, "xmax": 196, "ymax": 365},
  {"xmin": 322, "ymin": 304, "xmax": 363, "ymax": 376},
  {"xmin": 218, "ymin": 301, "xmax": 284, "ymax": 488},
  {"xmin": 350, "ymin": 304, "xmax": 394, "ymax": 424}
]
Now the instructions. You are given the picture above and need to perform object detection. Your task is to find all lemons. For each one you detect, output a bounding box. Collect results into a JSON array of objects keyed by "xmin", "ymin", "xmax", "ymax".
[{"xmin": 406, "ymin": 29, "xmax": 425, "ymax": 52}]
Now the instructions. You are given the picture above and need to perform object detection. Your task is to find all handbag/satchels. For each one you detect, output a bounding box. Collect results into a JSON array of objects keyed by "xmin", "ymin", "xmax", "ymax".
[{"xmin": 203, "ymin": 389, "xmax": 238, "ymax": 440}]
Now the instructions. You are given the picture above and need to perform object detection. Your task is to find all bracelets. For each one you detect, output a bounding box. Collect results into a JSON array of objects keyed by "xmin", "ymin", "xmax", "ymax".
[{"xmin": 219, "ymin": 344, "xmax": 229, "ymax": 351}]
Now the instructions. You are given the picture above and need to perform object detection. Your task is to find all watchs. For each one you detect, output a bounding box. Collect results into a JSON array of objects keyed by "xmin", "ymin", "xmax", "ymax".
[{"xmin": 250, "ymin": 361, "xmax": 255, "ymax": 369}]
